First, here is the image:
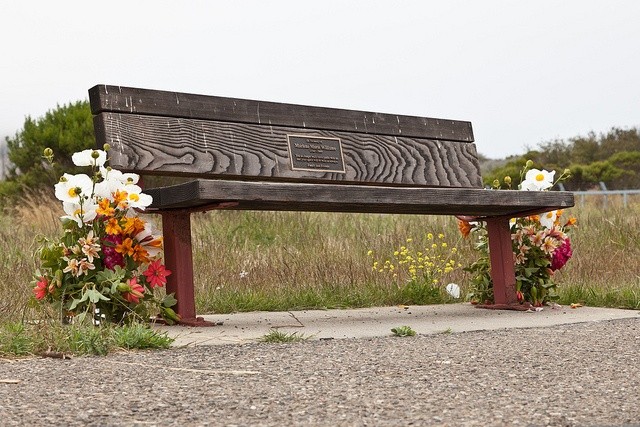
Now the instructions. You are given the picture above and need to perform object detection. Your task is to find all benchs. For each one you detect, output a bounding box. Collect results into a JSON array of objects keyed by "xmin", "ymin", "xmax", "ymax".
[{"xmin": 82, "ymin": 83, "xmax": 578, "ymax": 327}]
